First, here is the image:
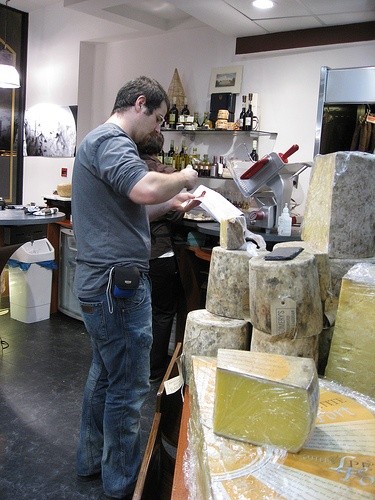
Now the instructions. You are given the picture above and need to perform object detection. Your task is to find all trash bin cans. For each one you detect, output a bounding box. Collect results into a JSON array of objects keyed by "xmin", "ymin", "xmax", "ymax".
[{"xmin": 8, "ymin": 238, "xmax": 55, "ymax": 324}]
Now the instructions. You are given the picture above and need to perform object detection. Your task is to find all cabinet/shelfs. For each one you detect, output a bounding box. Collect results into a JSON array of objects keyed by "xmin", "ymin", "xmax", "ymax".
[{"xmin": 157, "ymin": 128, "xmax": 277, "ymax": 181}]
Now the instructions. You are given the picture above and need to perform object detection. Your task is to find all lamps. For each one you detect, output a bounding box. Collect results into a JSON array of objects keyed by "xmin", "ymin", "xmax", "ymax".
[{"xmin": 0, "ymin": 0, "xmax": 21, "ymax": 88}]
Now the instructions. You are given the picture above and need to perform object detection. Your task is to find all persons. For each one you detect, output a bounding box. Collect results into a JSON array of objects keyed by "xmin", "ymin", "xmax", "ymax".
[
  {"xmin": 73, "ymin": 76, "xmax": 206, "ymax": 500},
  {"xmin": 136, "ymin": 131, "xmax": 185, "ymax": 382}
]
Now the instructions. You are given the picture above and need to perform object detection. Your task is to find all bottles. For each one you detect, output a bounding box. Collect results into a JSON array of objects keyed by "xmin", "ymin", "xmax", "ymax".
[
  {"xmin": 191, "ymin": 148, "xmax": 199, "ymax": 170},
  {"xmin": 243, "ymin": 93, "xmax": 253, "ymax": 131},
  {"xmin": 165, "ymin": 143, "xmax": 190, "ymax": 172},
  {"xmin": 210, "ymin": 156, "xmax": 218, "ymax": 178},
  {"xmin": 52, "ymin": 168, "xmax": 68, "ymax": 195},
  {"xmin": 158, "ymin": 149, "xmax": 165, "ymax": 164},
  {"xmin": 199, "ymin": 155, "xmax": 210, "ymax": 177},
  {"xmin": 239, "ymin": 95, "xmax": 246, "ymax": 129},
  {"xmin": 181, "ymin": 105, "xmax": 190, "ymax": 124},
  {"xmin": 168, "ymin": 104, "xmax": 178, "ymax": 125},
  {"xmin": 250, "ymin": 139, "xmax": 258, "ymax": 161},
  {"xmin": 218, "ymin": 157, "xmax": 224, "ymax": 177}
]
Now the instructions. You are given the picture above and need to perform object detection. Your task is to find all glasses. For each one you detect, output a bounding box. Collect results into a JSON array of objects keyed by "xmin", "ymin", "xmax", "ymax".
[{"xmin": 150, "ymin": 105, "xmax": 165, "ymax": 128}]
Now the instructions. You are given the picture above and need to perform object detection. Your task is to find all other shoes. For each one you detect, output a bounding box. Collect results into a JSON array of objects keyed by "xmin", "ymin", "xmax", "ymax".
[
  {"xmin": 76, "ymin": 471, "xmax": 101, "ymax": 482},
  {"xmin": 118, "ymin": 475, "xmax": 162, "ymax": 500},
  {"xmin": 149, "ymin": 372, "xmax": 164, "ymax": 383}
]
